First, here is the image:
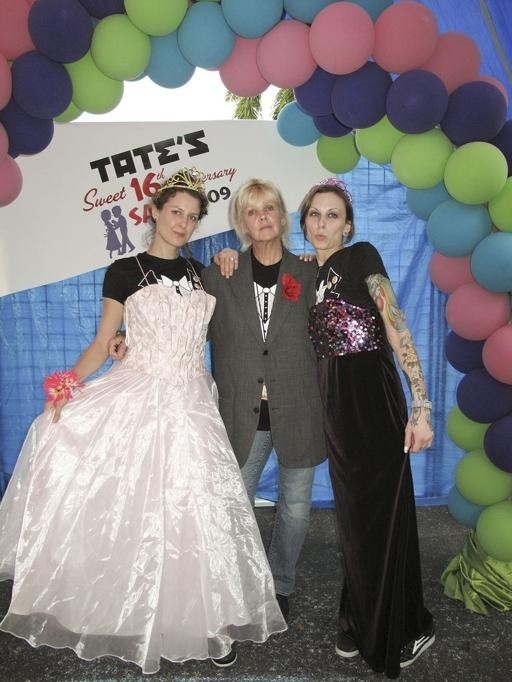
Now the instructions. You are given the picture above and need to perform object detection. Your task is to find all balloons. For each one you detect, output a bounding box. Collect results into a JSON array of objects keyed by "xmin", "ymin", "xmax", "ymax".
[
  {"xmin": 406, "ymin": 32, "xmax": 512, "ymax": 560},
  {"xmin": 2, "ymin": 0, "xmax": 452, "ymax": 206}
]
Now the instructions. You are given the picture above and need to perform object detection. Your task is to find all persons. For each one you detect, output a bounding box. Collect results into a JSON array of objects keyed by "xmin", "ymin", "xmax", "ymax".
[
  {"xmin": 107, "ymin": 178, "xmax": 327, "ymax": 667},
  {"xmin": 1, "ymin": 166, "xmax": 290, "ymax": 676},
  {"xmin": 298, "ymin": 180, "xmax": 437, "ymax": 679}
]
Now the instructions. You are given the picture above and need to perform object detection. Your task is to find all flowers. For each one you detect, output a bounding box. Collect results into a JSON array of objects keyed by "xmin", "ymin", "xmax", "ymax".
[
  {"xmin": 43, "ymin": 369, "xmax": 86, "ymax": 406},
  {"xmin": 281, "ymin": 272, "xmax": 301, "ymax": 303}
]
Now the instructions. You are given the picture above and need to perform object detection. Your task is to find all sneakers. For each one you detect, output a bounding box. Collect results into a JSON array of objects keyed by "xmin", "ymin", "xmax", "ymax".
[
  {"xmin": 394, "ymin": 618, "xmax": 438, "ymax": 670},
  {"xmin": 330, "ymin": 620, "xmax": 366, "ymax": 664},
  {"xmin": 274, "ymin": 589, "xmax": 291, "ymax": 631},
  {"xmin": 202, "ymin": 633, "xmax": 240, "ymax": 669}
]
